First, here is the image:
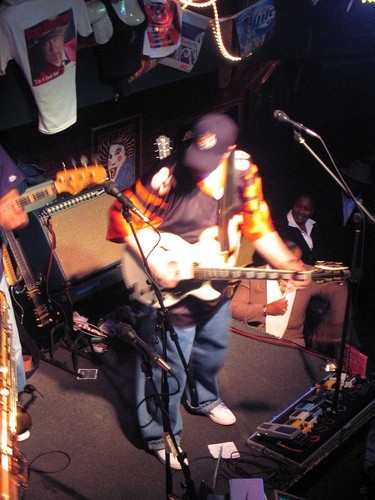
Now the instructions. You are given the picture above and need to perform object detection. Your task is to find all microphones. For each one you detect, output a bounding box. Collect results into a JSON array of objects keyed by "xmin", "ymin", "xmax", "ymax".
[
  {"xmin": 103, "ymin": 181, "xmax": 149, "ymax": 223},
  {"xmin": 273, "ymin": 109, "xmax": 321, "ymax": 138},
  {"xmin": 114, "ymin": 323, "xmax": 171, "ymax": 373}
]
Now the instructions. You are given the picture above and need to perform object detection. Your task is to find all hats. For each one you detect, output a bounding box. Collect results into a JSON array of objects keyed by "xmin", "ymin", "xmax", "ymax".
[
  {"xmin": 27, "ymin": 19, "xmax": 70, "ymax": 52},
  {"xmin": 110, "ymin": 0, "xmax": 145, "ymax": 26},
  {"xmin": 86, "ymin": 0, "xmax": 113, "ymax": 45},
  {"xmin": 185, "ymin": 113, "xmax": 237, "ymax": 172}
]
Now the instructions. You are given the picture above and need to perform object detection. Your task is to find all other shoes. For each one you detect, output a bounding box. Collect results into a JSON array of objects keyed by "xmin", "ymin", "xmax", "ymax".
[
  {"xmin": 206, "ymin": 401, "xmax": 236, "ymax": 425},
  {"xmin": 155, "ymin": 445, "xmax": 189, "ymax": 470}
]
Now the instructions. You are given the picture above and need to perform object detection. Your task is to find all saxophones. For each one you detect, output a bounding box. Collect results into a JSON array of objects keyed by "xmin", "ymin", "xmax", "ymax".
[{"xmin": 0, "ymin": 291, "xmax": 27, "ymax": 500}]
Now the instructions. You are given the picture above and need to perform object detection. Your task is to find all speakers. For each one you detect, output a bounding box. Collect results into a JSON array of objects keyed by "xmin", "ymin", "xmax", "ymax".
[{"xmin": 20, "ymin": 184, "xmax": 123, "ymax": 306}]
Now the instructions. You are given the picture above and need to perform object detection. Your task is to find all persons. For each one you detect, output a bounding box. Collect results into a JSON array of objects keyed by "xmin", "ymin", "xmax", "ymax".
[
  {"xmin": 0, "ymin": 144, "xmax": 31, "ymax": 442},
  {"xmin": 274, "ymin": 195, "xmax": 336, "ymax": 262},
  {"xmin": 315, "ymin": 168, "xmax": 373, "ymax": 282},
  {"xmin": 108, "ymin": 113, "xmax": 314, "ymax": 470},
  {"xmin": 232, "ymin": 240, "xmax": 349, "ymax": 349},
  {"xmin": 30, "ymin": 18, "xmax": 76, "ymax": 84}
]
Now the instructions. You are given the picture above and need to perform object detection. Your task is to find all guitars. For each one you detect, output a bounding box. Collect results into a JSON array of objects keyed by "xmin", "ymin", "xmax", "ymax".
[
  {"xmin": 1, "ymin": 153, "xmax": 109, "ymax": 215},
  {"xmin": 121, "ymin": 230, "xmax": 350, "ymax": 310},
  {"xmin": 2, "ymin": 224, "xmax": 68, "ymax": 353}
]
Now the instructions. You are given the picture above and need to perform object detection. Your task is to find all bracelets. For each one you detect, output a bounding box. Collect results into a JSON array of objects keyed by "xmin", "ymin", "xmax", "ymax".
[{"xmin": 263, "ymin": 304, "xmax": 268, "ymax": 317}]
